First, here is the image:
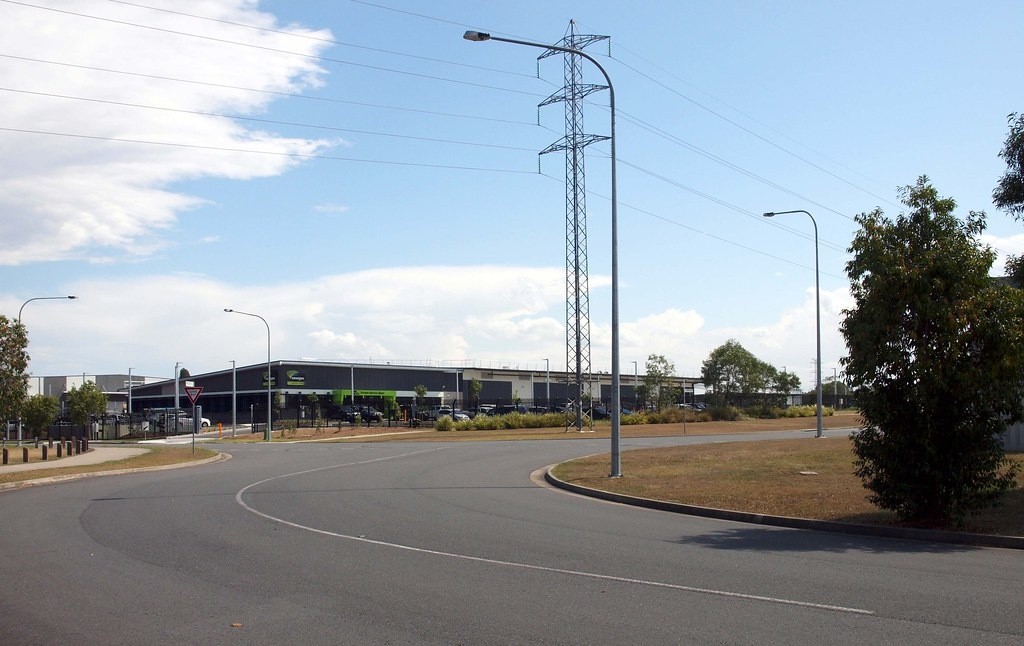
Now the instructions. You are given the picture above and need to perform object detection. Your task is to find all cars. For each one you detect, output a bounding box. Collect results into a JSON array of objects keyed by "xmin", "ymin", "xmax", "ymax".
[
  {"xmin": 434, "ymin": 408, "xmax": 470, "ymax": 422},
  {"xmin": 459, "ymin": 400, "xmax": 711, "ymax": 421},
  {"xmin": 159, "ymin": 412, "xmax": 212, "ymax": 435},
  {"xmin": 351, "ymin": 405, "xmax": 384, "ymax": 422},
  {"xmin": 53, "ymin": 405, "xmax": 188, "ymax": 426},
  {"xmin": 324, "ymin": 404, "xmax": 361, "ymax": 421},
  {"xmin": 2, "ymin": 419, "xmax": 26, "ymax": 432}
]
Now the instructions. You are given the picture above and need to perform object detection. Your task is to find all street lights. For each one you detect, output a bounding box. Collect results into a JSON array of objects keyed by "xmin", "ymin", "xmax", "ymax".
[
  {"xmin": 763, "ymin": 209, "xmax": 824, "ymax": 438},
  {"xmin": 5, "ymin": 294, "xmax": 79, "ymax": 441},
  {"xmin": 223, "ymin": 308, "xmax": 273, "ymax": 442},
  {"xmin": 462, "ymin": 29, "xmax": 622, "ymax": 478}
]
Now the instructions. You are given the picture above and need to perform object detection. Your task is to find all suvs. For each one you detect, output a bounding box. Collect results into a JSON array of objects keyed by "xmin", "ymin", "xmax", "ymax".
[{"xmin": 417, "ymin": 404, "xmax": 451, "ymax": 421}]
